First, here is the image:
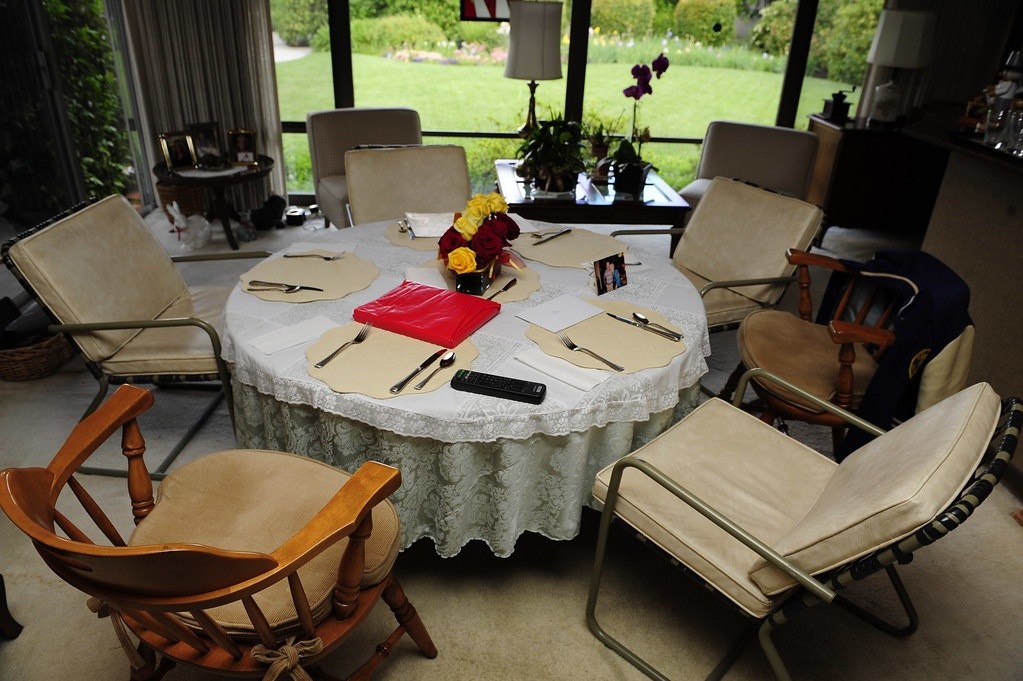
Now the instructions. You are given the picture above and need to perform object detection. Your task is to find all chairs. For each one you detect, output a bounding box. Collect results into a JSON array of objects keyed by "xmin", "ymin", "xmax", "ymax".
[
  {"xmin": 584, "ymin": 379, "xmax": 1023, "ymax": 681},
  {"xmin": 682, "ymin": 122, "xmax": 819, "ymax": 207},
  {"xmin": 0, "ymin": 380, "xmax": 440, "ymax": 681},
  {"xmin": 1, "ymin": 186, "xmax": 246, "ymax": 481},
  {"xmin": 672, "ymin": 177, "xmax": 824, "ymax": 334},
  {"xmin": 695, "ymin": 248, "xmax": 910, "ymax": 452},
  {"xmin": 308, "ymin": 109, "xmax": 422, "ymax": 234},
  {"xmin": 344, "ymin": 146, "xmax": 471, "ymax": 226}
]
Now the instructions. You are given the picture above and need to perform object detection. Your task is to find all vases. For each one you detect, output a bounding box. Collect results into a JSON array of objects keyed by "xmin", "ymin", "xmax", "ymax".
[
  {"xmin": 449, "ymin": 257, "xmax": 502, "ymax": 296},
  {"xmin": 615, "ymin": 162, "xmax": 652, "ymax": 195}
]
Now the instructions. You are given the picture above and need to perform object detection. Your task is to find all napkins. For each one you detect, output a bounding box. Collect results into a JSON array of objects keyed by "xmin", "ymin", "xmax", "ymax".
[
  {"xmin": 248, "ymin": 314, "xmax": 343, "ymax": 356},
  {"xmin": 507, "ymin": 211, "xmax": 537, "ymax": 238},
  {"xmin": 515, "ymin": 349, "xmax": 610, "ymax": 391},
  {"xmin": 585, "ymin": 253, "xmax": 650, "ymax": 277},
  {"xmin": 285, "ymin": 241, "xmax": 360, "ymax": 258}
]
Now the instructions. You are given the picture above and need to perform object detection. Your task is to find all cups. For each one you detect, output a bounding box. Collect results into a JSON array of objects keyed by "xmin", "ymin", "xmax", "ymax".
[{"xmin": 962, "ymin": 102, "xmax": 1023, "ymax": 157}]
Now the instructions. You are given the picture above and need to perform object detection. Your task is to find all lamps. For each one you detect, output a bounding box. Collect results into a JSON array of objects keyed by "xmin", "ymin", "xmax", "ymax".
[
  {"xmin": 863, "ymin": 7, "xmax": 938, "ymax": 122},
  {"xmin": 504, "ymin": 3, "xmax": 564, "ymax": 136}
]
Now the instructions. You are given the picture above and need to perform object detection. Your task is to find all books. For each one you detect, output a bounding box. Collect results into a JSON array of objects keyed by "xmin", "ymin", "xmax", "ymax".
[{"xmin": 404, "ymin": 212, "xmax": 541, "ymax": 238}]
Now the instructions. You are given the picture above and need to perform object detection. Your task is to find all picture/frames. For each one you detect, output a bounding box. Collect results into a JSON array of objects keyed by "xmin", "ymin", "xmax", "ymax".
[
  {"xmin": 158, "ymin": 131, "xmax": 189, "ymax": 173},
  {"xmin": 227, "ymin": 130, "xmax": 260, "ymax": 166},
  {"xmin": 185, "ymin": 119, "xmax": 224, "ymax": 170}
]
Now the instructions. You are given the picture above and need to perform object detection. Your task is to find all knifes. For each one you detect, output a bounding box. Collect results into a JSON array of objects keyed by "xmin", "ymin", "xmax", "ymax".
[
  {"xmin": 404, "ymin": 217, "xmax": 414, "ymax": 240},
  {"xmin": 249, "ymin": 281, "xmax": 324, "ymax": 291},
  {"xmin": 390, "ymin": 349, "xmax": 448, "ymax": 394},
  {"xmin": 533, "ymin": 230, "xmax": 572, "ymax": 246},
  {"xmin": 487, "ymin": 278, "xmax": 516, "ymax": 301},
  {"xmin": 607, "ymin": 313, "xmax": 679, "ymax": 342}
]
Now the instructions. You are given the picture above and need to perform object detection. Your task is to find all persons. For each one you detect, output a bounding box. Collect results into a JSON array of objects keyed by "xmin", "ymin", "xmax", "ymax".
[
  {"xmin": 195, "ymin": 133, "xmax": 212, "ymax": 157},
  {"xmin": 172, "ymin": 139, "xmax": 186, "ymax": 160},
  {"xmin": 604, "ymin": 262, "xmax": 620, "ymax": 291},
  {"xmin": 231, "ymin": 134, "xmax": 251, "ymax": 161}
]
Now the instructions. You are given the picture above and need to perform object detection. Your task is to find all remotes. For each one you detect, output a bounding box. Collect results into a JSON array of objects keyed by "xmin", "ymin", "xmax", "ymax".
[{"xmin": 451, "ymin": 369, "xmax": 546, "ymax": 405}]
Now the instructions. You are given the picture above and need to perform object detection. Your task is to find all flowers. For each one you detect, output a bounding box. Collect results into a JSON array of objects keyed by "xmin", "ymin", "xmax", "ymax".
[
  {"xmin": 438, "ymin": 191, "xmax": 524, "ymax": 272},
  {"xmin": 597, "ymin": 55, "xmax": 670, "ymax": 177}
]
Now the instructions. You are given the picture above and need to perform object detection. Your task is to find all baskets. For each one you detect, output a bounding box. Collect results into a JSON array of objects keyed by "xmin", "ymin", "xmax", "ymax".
[{"xmin": 0, "ymin": 332, "xmax": 72, "ymax": 381}]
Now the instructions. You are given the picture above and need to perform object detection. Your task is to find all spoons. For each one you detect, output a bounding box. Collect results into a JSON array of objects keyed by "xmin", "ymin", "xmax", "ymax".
[
  {"xmin": 397, "ymin": 220, "xmax": 406, "ymax": 232},
  {"xmin": 247, "ymin": 286, "xmax": 300, "ymax": 293},
  {"xmin": 533, "ymin": 232, "xmax": 560, "ymax": 237},
  {"xmin": 633, "ymin": 312, "xmax": 684, "ymax": 339},
  {"xmin": 414, "ymin": 352, "xmax": 456, "ymax": 390}
]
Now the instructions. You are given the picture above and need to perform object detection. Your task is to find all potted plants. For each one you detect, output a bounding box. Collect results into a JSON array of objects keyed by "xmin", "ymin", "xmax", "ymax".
[{"xmin": 515, "ymin": 110, "xmax": 586, "ymax": 195}]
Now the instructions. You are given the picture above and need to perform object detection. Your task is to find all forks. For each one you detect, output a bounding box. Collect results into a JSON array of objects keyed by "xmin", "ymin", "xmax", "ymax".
[
  {"xmin": 558, "ymin": 331, "xmax": 625, "ymax": 372},
  {"xmin": 314, "ymin": 322, "xmax": 372, "ymax": 368},
  {"xmin": 283, "ymin": 251, "xmax": 345, "ymax": 261}
]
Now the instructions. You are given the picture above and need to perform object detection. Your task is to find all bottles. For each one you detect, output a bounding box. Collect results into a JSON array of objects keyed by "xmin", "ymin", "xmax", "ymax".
[{"xmin": 870, "ymin": 80, "xmax": 904, "ymax": 122}]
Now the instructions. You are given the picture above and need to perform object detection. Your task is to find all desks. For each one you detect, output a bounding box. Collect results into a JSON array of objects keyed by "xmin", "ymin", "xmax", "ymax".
[
  {"xmin": 222, "ymin": 234, "xmax": 713, "ymax": 560},
  {"xmin": 801, "ymin": 112, "xmax": 925, "ymax": 229},
  {"xmin": 153, "ymin": 153, "xmax": 276, "ymax": 250},
  {"xmin": 495, "ymin": 155, "xmax": 690, "ymax": 231}
]
